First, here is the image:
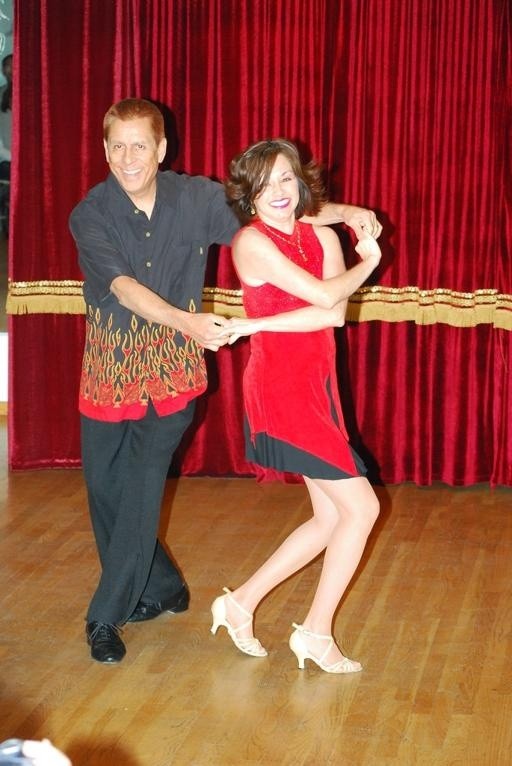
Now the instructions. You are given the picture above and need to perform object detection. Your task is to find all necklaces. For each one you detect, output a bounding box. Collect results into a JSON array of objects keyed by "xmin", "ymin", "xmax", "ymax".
[{"xmin": 261, "ymin": 222, "xmax": 310, "ymax": 265}]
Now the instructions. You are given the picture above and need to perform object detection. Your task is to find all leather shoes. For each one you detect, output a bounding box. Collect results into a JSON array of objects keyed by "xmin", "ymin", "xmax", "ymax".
[
  {"xmin": 126, "ymin": 584, "xmax": 190, "ymax": 623},
  {"xmin": 87, "ymin": 620, "xmax": 126, "ymax": 664}
]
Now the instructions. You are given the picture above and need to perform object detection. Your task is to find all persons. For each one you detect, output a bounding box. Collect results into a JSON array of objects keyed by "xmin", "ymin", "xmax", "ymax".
[
  {"xmin": 69, "ymin": 100, "xmax": 383, "ymax": 663},
  {"xmin": 1, "ymin": 56, "xmax": 12, "ymax": 238},
  {"xmin": 210, "ymin": 139, "xmax": 382, "ymax": 676}
]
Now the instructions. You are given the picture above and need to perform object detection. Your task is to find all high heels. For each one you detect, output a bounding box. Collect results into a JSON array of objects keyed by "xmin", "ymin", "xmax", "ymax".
[
  {"xmin": 287, "ymin": 620, "xmax": 363, "ymax": 673},
  {"xmin": 211, "ymin": 584, "xmax": 267, "ymax": 656}
]
